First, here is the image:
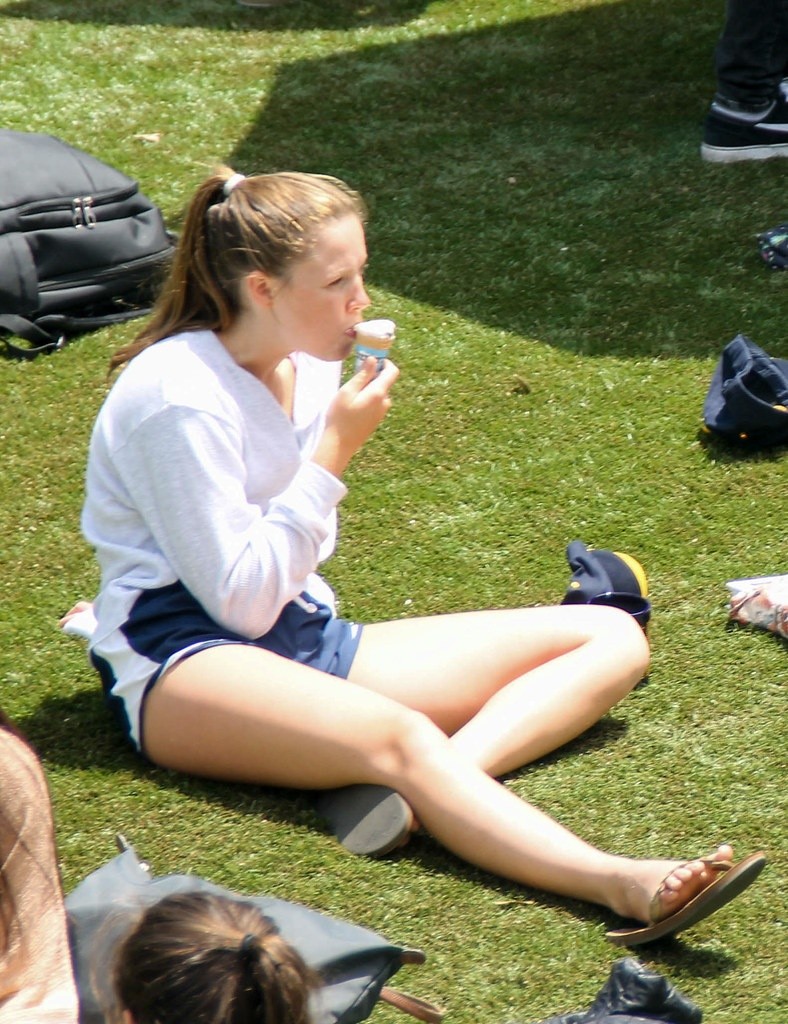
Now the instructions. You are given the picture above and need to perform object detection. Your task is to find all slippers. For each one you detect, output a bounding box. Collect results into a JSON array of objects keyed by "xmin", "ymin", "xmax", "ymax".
[
  {"xmin": 313, "ymin": 781, "xmax": 423, "ymax": 860},
  {"xmin": 605, "ymin": 851, "xmax": 767, "ymax": 947}
]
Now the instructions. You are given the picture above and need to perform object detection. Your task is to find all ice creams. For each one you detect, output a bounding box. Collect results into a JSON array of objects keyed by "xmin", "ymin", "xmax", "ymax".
[{"xmin": 353, "ymin": 318, "xmax": 396, "ymax": 351}]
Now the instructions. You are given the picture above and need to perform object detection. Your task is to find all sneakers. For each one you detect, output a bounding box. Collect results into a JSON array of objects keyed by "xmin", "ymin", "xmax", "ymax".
[{"xmin": 701, "ymin": 82, "xmax": 788, "ymax": 163}]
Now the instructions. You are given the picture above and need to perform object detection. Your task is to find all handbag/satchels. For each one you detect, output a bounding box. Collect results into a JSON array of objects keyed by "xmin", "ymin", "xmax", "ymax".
[
  {"xmin": 727, "ymin": 574, "xmax": 788, "ymax": 640},
  {"xmin": 62, "ymin": 831, "xmax": 445, "ymax": 1024},
  {"xmin": 703, "ymin": 332, "xmax": 788, "ymax": 452},
  {"xmin": 0, "ymin": 128, "xmax": 180, "ymax": 358}
]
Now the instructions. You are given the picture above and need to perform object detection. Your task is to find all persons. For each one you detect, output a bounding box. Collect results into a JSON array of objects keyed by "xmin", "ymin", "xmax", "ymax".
[
  {"xmin": 57, "ymin": 166, "xmax": 764, "ymax": 946},
  {"xmin": 0, "ymin": 706, "xmax": 80, "ymax": 1024},
  {"xmin": 701, "ymin": 0, "xmax": 788, "ymax": 163},
  {"xmin": 89, "ymin": 890, "xmax": 319, "ymax": 1024}
]
